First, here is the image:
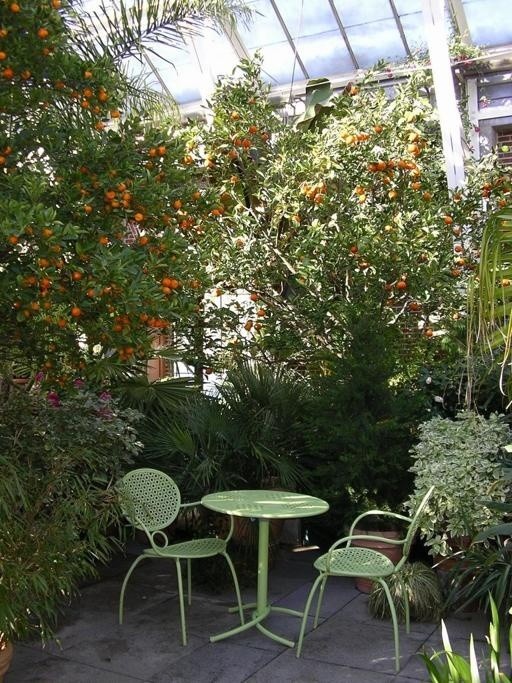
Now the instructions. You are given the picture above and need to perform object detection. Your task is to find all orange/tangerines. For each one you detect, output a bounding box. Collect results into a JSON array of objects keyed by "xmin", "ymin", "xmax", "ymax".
[{"xmin": 0, "ymin": 0, "xmax": 512, "ymax": 389}]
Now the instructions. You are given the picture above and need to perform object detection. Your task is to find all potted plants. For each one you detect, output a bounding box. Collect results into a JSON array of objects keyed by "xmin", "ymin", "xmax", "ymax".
[
  {"xmin": 403, "ymin": 410, "xmax": 512, "ymax": 613},
  {"xmin": 0, "ymin": 429, "xmax": 152, "ymax": 677}
]
